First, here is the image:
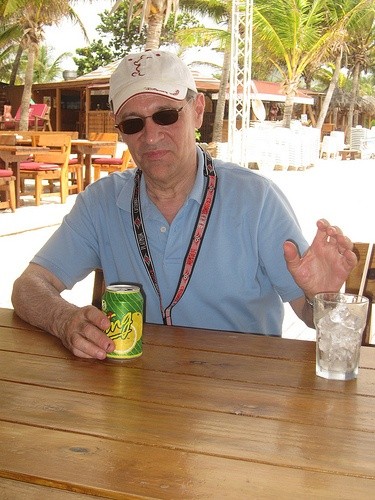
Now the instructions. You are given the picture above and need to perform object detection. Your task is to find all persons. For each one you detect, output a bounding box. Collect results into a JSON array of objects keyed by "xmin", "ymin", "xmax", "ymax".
[{"xmin": 10, "ymin": 50, "xmax": 357, "ymax": 360}]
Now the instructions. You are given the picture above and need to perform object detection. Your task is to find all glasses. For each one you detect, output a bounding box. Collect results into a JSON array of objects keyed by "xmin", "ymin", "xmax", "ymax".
[{"xmin": 115, "ymin": 97, "xmax": 193, "ymax": 134}]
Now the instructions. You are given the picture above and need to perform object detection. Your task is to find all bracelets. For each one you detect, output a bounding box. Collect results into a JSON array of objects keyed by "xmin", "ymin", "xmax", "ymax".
[{"xmin": 304, "ymin": 297, "xmax": 314, "ymax": 308}]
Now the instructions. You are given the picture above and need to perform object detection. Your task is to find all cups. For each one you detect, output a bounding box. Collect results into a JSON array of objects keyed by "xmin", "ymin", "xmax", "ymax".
[{"xmin": 313, "ymin": 292, "xmax": 369, "ymax": 381}]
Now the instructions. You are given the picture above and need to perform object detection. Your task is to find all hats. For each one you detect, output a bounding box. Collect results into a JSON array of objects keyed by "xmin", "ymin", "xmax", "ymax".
[{"xmin": 108, "ymin": 50, "xmax": 197, "ymax": 116}]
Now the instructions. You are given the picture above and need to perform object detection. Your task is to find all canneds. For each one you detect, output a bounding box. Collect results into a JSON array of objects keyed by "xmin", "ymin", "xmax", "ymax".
[{"xmin": 102, "ymin": 285, "xmax": 144, "ymax": 361}]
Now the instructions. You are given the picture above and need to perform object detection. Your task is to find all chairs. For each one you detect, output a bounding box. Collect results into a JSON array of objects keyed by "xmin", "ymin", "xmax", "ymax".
[{"xmin": 0, "ymin": 132, "xmax": 137, "ymax": 212}]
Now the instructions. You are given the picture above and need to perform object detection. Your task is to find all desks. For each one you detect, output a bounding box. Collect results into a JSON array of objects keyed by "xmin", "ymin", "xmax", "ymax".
[
  {"xmin": 0, "ymin": 147, "xmax": 47, "ymax": 208},
  {"xmin": 0, "ymin": 307, "xmax": 375, "ymax": 500},
  {"xmin": 16, "ymin": 141, "xmax": 116, "ymax": 190}
]
